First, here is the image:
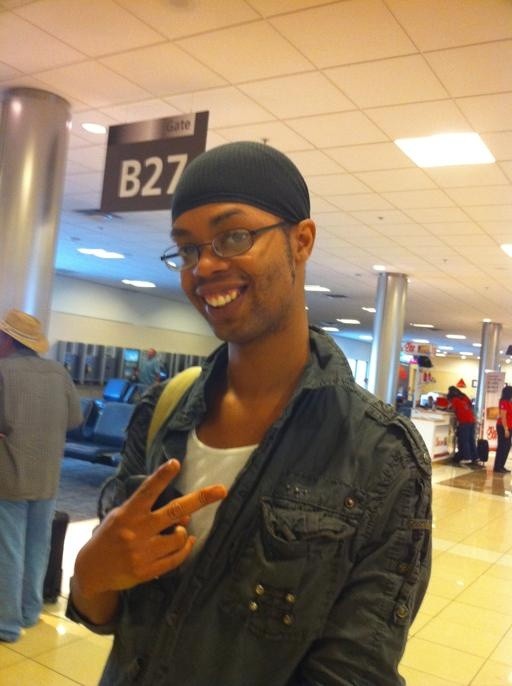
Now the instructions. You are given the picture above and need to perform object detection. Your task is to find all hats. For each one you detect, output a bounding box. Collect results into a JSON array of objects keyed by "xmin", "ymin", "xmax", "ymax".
[
  {"xmin": 171, "ymin": 141, "xmax": 311, "ymax": 224},
  {"xmin": 0, "ymin": 307, "xmax": 50, "ymax": 354}
]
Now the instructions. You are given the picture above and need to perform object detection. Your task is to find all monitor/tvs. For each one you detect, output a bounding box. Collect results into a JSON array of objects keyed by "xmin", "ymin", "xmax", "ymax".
[
  {"xmin": 124, "ymin": 348, "xmax": 139, "ymax": 362},
  {"xmin": 420, "ymin": 393, "xmax": 439, "ymax": 406}
]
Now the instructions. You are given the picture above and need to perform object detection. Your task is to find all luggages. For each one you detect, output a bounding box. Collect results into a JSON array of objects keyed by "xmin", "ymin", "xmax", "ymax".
[{"xmin": 477, "ymin": 439, "xmax": 489, "ymax": 462}]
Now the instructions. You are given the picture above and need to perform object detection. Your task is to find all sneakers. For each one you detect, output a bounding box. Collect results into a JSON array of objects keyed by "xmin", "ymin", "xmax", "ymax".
[
  {"xmin": 460, "ymin": 459, "xmax": 473, "ymax": 463},
  {"xmin": 493, "ymin": 467, "xmax": 511, "ymax": 473}
]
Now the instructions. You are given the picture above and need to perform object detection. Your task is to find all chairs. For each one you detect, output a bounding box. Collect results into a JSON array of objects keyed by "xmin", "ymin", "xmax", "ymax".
[{"xmin": 64, "ymin": 379, "xmax": 138, "ymax": 467}]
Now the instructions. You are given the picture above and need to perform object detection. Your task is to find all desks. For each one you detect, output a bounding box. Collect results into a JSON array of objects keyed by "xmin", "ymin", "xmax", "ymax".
[{"xmin": 410, "ymin": 407, "xmax": 459, "ymax": 463}]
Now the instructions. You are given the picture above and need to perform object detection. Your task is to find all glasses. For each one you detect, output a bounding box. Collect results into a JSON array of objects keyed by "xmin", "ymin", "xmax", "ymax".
[{"xmin": 160, "ymin": 219, "xmax": 284, "ymax": 274}]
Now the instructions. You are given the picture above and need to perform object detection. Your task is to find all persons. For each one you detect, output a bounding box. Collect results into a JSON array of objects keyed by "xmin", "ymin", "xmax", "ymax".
[
  {"xmin": 447, "ymin": 384, "xmax": 479, "ymax": 473},
  {"xmin": 66, "ymin": 138, "xmax": 434, "ymax": 684},
  {"xmin": 130, "ymin": 346, "xmax": 163, "ymax": 387},
  {"xmin": 2, "ymin": 305, "xmax": 86, "ymax": 640},
  {"xmin": 494, "ymin": 385, "xmax": 512, "ymax": 472}
]
